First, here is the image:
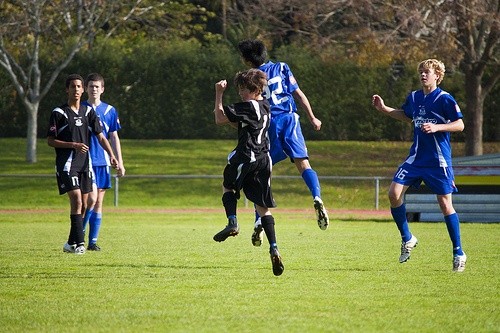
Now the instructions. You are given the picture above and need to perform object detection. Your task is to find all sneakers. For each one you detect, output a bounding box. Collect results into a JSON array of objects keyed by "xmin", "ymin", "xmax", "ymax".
[
  {"xmin": 398, "ymin": 232, "xmax": 418, "ymax": 263},
  {"xmin": 251, "ymin": 218, "xmax": 264, "ymax": 247},
  {"xmin": 270, "ymin": 247, "xmax": 285, "ymax": 277},
  {"xmin": 213, "ymin": 225, "xmax": 239, "ymax": 242},
  {"xmin": 313, "ymin": 197, "xmax": 330, "ymax": 231},
  {"xmin": 88, "ymin": 242, "xmax": 101, "ymax": 252},
  {"xmin": 452, "ymin": 251, "xmax": 467, "ymax": 272},
  {"xmin": 63, "ymin": 241, "xmax": 86, "ymax": 255}
]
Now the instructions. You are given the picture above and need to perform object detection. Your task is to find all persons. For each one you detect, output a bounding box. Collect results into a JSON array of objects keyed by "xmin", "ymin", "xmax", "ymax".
[
  {"xmin": 213, "ymin": 68, "xmax": 284, "ymax": 276},
  {"xmin": 239, "ymin": 39, "xmax": 330, "ymax": 247},
  {"xmin": 82, "ymin": 72, "xmax": 126, "ymax": 251},
  {"xmin": 47, "ymin": 74, "xmax": 119, "ymax": 253},
  {"xmin": 371, "ymin": 59, "xmax": 467, "ymax": 272}
]
